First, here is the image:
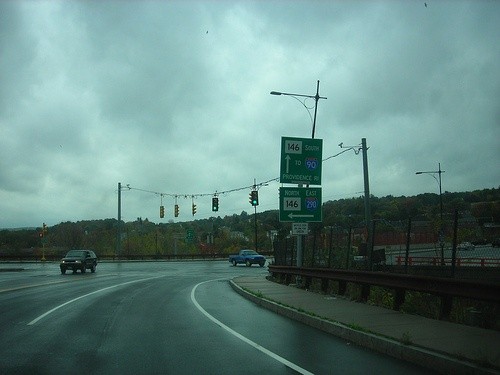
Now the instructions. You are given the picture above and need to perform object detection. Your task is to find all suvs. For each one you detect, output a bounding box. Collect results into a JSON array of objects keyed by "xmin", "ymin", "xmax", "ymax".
[{"xmin": 59, "ymin": 249, "xmax": 98, "ymax": 274}]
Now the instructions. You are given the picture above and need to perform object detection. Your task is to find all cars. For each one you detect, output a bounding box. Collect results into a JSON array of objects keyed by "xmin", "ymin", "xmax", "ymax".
[{"xmin": 457, "ymin": 242, "xmax": 475, "ymax": 251}]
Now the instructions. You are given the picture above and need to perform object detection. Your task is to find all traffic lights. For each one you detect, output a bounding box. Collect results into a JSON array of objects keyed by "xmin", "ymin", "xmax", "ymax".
[
  {"xmin": 249, "ymin": 193, "xmax": 252, "ymax": 204},
  {"xmin": 160, "ymin": 206, "xmax": 164, "ymax": 218},
  {"xmin": 39, "ymin": 232, "xmax": 43, "ymax": 237},
  {"xmin": 43, "ymin": 223, "xmax": 47, "ymax": 233},
  {"xmin": 192, "ymin": 203, "xmax": 196, "ymax": 216},
  {"xmin": 174, "ymin": 205, "xmax": 180, "ymax": 217},
  {"xmin": 251, "ymin": 191, "xmax": 259, "ymax": 206},
  {"xmin": 212, "ymin": 198, "xmax": 218, "ymax": 211}
]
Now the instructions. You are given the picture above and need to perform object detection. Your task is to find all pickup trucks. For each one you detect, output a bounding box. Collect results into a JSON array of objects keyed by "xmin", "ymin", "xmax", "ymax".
[{"xmin": 228, "ymin": 249, "xmax": 266, "ymax": 268}]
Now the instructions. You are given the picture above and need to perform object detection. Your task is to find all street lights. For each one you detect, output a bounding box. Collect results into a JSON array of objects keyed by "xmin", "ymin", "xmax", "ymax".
[
  {"xmin": 415, "ymin": 161, "xmax": 445, "ymax": 268},
  {"xmin": 269, "ymin": 79, "xmax": 328, "ymax": 289}
]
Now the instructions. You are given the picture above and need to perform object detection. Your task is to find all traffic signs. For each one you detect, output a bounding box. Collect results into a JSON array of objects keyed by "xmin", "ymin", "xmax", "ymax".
[
  {"xmin": 278, "ymin": 186, "xmax": 323, "ymax": 223},
  {"xmin": 279, "ymin": 136, "xmax": 323, "ymax": 186}
]
